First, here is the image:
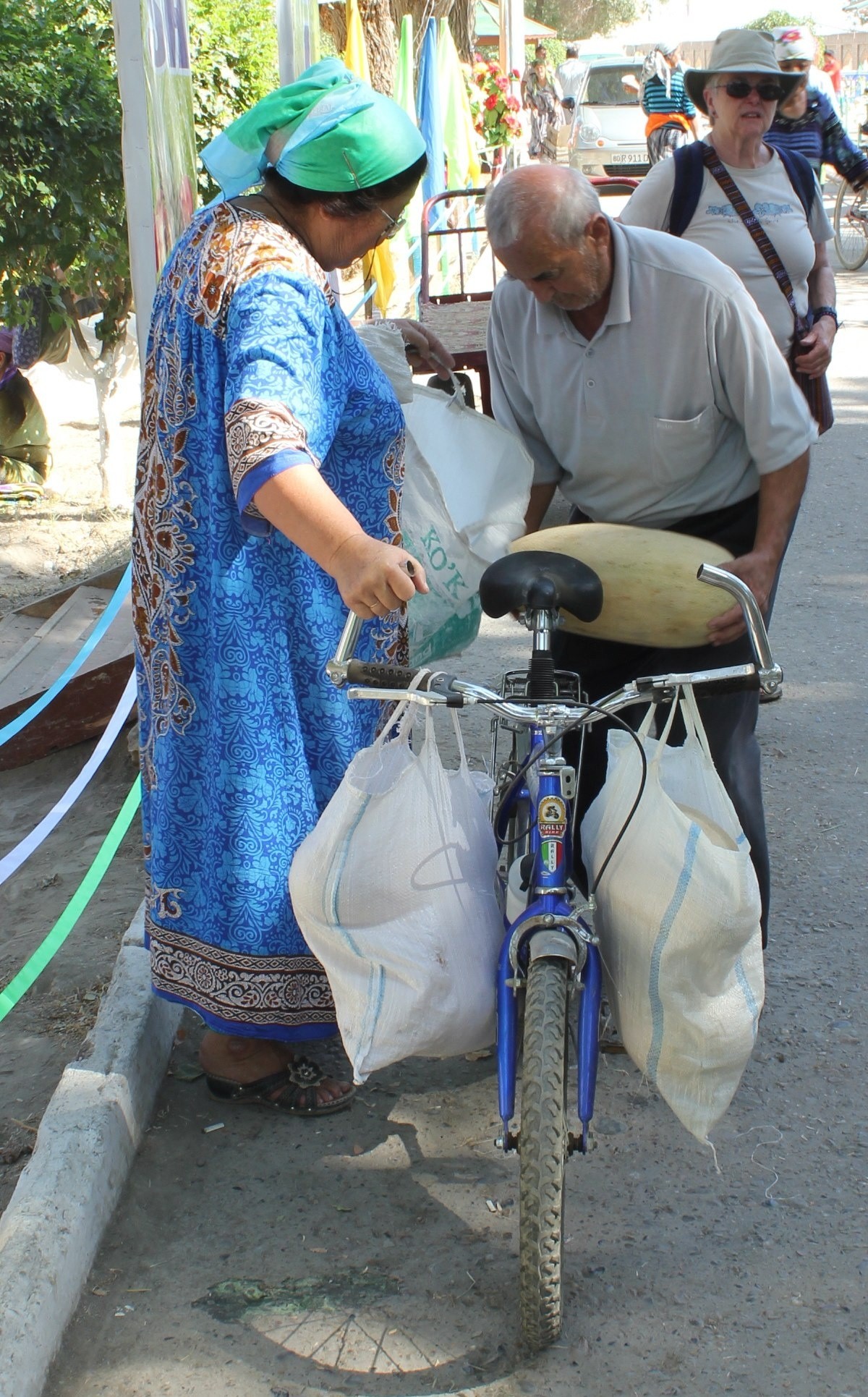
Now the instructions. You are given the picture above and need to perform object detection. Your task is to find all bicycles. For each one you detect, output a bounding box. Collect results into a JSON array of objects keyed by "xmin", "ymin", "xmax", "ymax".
[
  {"xmin": 324, "ymin": 551, "xmax": 789, "ymax": 1353},
  {"xmin": 832, "ymin": 145, "xmax": 868, "ymax": 271}
]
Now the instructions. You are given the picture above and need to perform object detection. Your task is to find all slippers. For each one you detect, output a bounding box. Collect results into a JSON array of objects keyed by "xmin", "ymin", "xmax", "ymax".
[{"xmin": 206, "ymin": 1054, "xmax": 357, "ymax": 1116}]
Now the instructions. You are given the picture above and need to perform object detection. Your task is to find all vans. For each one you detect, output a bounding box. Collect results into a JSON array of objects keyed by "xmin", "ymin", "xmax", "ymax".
[{"xmin": 560, "ymin": 56, "xmax": 693, "ymax": 181}]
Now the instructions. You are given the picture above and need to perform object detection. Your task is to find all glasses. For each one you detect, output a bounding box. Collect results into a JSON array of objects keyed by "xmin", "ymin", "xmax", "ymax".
[
  {"xmin": 706, "ymin": 82, "xmax": 787, "ymax": 103},
  {"xmin": 360, "ymin": 188, "xmax": 405, "ymax": 238}
]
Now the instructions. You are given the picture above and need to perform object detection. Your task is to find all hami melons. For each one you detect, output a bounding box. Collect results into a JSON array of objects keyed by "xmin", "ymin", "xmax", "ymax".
[
  {"xmin": 675, "ymin": 800, "xmax": 740, "ymax": 851},
  {"xmin": 509, "ymin": 522, "xmax": 736, "ymax": 647}
]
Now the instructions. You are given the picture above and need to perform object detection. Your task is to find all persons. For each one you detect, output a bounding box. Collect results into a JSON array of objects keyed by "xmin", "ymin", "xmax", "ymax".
[
  {"xmin": 613, "ymin": 29, "xmax": 844, "ymax": 437},
  {"xmin": 555, "ymin": 45, "xmax": 588, "ymax": 127},
  {"xmin": 763, "ymin": 25, "xmax": 868, "ymax": 184},
  {"xmin": 640, "ymin": 40, "xmax": 700, "ymax": 167},
  {"xmin": 485, "ymin": 164, "xmax": 820, "ymax": 951},
  {"xmin": 0, "ymin": 325, "xmax": 55, "ymax": 500},
  {"xmin": 132, "ymin": 54, "xmax": 455, "ymax": 1118},
  {"xmin": 809, "ymin": 36, "xmax": 844, "ymax": 192},
  {"xmin": 521, "ymin": 45, "xmax": 566, "ymax": 159},
  {"xmin": 821, "ymin": 50, "xmax": 842, "ymax": 109}
]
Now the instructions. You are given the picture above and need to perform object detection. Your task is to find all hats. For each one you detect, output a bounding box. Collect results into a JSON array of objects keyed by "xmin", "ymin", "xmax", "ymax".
[
  {"xmin": 683, "ymin": 27, "xmax": 807, "ymax": 116},
  {"xmin": 769, "ymin": 26, "xmax": 815, "ymax": 62}
]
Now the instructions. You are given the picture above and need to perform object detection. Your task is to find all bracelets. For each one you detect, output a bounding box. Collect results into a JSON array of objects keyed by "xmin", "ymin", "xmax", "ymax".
[{"xmin": 812, "ymin": 306, "xmax": 843, "ymax": 333}]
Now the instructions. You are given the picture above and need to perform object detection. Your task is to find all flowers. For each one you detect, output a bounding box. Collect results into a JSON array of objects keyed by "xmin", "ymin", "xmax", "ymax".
[{"xmin": 470, "ymin": 58, "xmax": 522, "ymax": 144}]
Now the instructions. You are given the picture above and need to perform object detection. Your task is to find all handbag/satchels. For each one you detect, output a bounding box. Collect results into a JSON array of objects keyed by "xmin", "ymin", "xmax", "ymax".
[
  {"xmin": 580, "ymin": 674, "xmax": 765, "ymax": 1145},
  {"xmin": 398, "ymin": 348, "xmax": 536, "ymax": 668},
  {"xmin": 286, "ymin": 671, "xmax": 506, "ymax": 1086},
  {"xmin": 789, "ymin": 320, "xmax": 834, "ymax": 436}
]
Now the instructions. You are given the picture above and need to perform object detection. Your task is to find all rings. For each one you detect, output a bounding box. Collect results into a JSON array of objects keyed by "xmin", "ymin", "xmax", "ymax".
[{"xmin": 370, "ymin": 600, "xmax": 379, "ymax": 608}]
